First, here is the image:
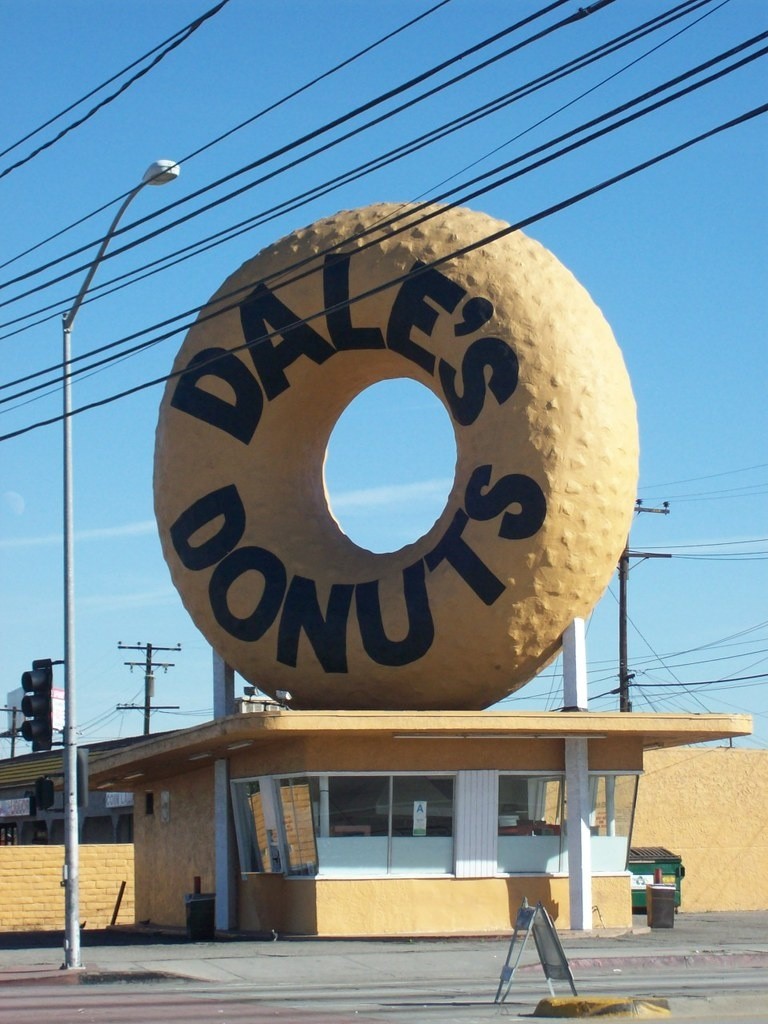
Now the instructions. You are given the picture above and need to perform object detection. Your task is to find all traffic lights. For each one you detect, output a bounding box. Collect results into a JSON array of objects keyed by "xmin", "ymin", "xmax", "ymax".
[{"xmin": 21, "ymin": 658, "xmax": 52, "ymax": 753}]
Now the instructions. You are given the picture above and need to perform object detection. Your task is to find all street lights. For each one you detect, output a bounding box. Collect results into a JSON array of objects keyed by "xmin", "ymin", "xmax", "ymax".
[{"xmin": 60, "ymin": 157, "xmax": 181, "ymax": 970}]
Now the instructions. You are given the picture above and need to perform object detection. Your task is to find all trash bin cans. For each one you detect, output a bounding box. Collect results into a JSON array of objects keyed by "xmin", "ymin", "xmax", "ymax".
[
  {"xmin": 646, "ymin": 883, "xmax": 674, "ymax": 928},
  {"xmin": 628, "ymin": 846, "xmax": 685, "ymax": 909},
  {"xmin": 186, "ymin": 893, "xmax": 215, "ymax": 940}
]
